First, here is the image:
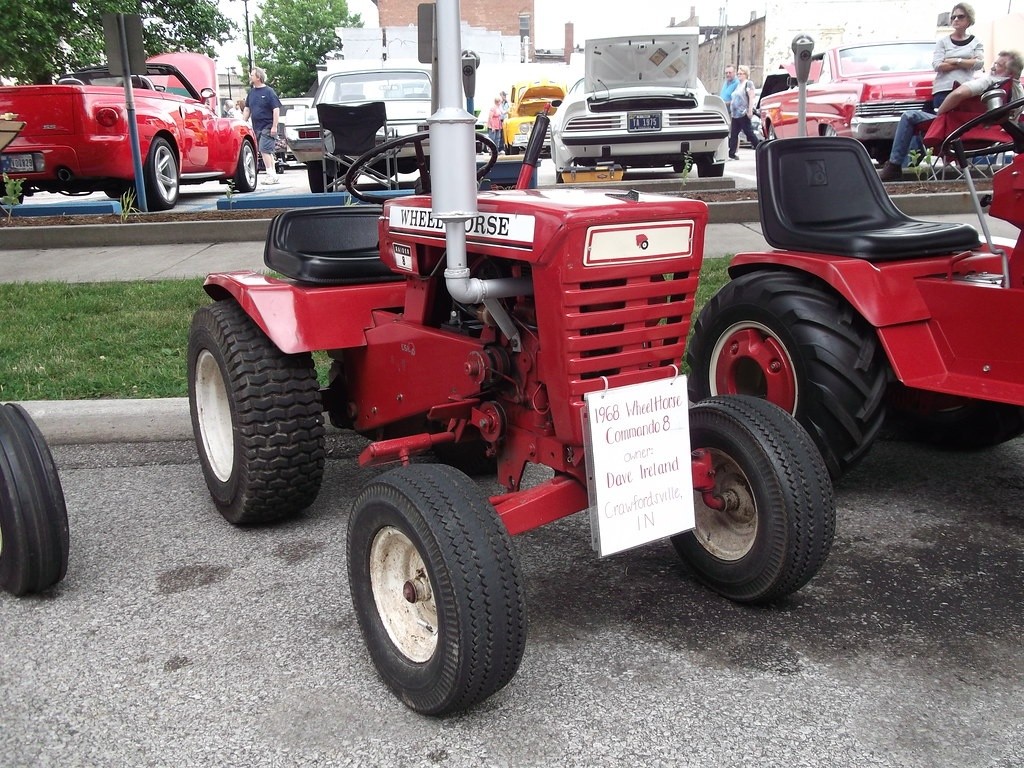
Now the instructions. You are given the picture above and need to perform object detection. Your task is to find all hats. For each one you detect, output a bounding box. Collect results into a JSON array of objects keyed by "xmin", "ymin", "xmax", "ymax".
[{"xmin": 499, "ymin": 91, "xmax": 508, "ymax": 96}]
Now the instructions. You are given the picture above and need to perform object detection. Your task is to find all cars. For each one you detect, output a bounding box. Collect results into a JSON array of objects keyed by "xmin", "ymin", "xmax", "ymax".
[
  {"xmin": 499, "ymin": 77, "xmax": 569, "ymax": 155},
  {"xmin": 283, "ymin": 66, "xmax": 434, "ymax": 194},
  {"xmin": 0, "ymin": 62, "xmax": 260, "ymax": 209},
  {"xmin": 274, "ymin": 97, "xmax": 315, "ymax": 160},
  {"xmin": 548, "ymin": 23, "xmax": 732, "ymax": 186},
  {"xmin": 759, "ymin": 38, "xmax": 945, "ymax": 163}
]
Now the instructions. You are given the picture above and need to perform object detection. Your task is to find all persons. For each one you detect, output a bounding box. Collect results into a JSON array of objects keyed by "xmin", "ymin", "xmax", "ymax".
[
  {"xmin": 720, "ymin": 64, "xmax": 761, "ymax": 161},
  {"xmin": 487, "ymin": 90, "xmax": 511, "ymax": 155},
  {"xmin": 877, "ymin": 3, "xmax": 1024, "ymax": 182},
  {"xmin": 223, "ymin": 66, "xmax": 282, "ymax": 186}
]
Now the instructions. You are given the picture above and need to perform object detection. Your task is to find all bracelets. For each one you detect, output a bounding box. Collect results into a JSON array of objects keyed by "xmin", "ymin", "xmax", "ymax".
[{"xmin": 957, "ymin": 58, "xmax": 961, "ymax": 64}]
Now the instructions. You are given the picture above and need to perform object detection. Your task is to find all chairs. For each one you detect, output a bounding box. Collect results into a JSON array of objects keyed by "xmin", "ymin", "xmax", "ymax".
[
  {"xmin": 122, "ymin": 75, "xmax": 157, "ymax": 90},
  {"xmin": 756, "ymin": 136, "xmax": 981, "ymax": 261},
  {"xmin": 263, "ymin": 205, "xmax": 404, "ymax": 283},
  {"xmin": 314, "ymin": 100, "xmax": 402, "ymax": 192},
  {"xmin": 59, "ymin": 78, "xmax": 85, "ymax": 85}
]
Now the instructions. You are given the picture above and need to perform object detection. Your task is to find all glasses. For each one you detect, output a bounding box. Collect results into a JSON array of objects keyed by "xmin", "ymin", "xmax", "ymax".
[
  {"xmin": 737, "ymin": 73, "xmax": 746, "ymax": 76},
  {"xmin": 951, "ymin": 14, "xmax": 967, "ymax": 20}
]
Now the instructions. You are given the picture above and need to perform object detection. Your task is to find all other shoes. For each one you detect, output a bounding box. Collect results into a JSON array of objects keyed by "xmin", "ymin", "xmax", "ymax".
[
  {"xmin": 879, "ymin": 160, "xmax": 904, "ymax": 182},
  {"xmin": 261, "ymin": 174, "xmax": 279, "ymax": 184},
  {"xmin": 728, "ymin": 155, "xmax": 739, "ymax": 161},
  {"xmin": 500, "ymin": 150, "xmax": 505, "ymax": 154}
]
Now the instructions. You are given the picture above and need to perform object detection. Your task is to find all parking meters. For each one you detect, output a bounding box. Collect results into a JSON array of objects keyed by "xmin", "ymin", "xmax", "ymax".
[
  {"xmin": 791, "ymin": 35, "xmax": 813, "ymax": 138},
  {"xmin": 462, "ymin": 50, "xmax": 479, "ymax": 115}
]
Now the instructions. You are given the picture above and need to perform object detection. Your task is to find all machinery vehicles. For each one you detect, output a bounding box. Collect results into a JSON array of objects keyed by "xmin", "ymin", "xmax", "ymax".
[
  {"xmin": 691, "ymin": 94, "xmax": 1024, "ymax": 485},
  {"xmin": 187, "ymin": 0, "xmax": 839, "ymax": 716}
]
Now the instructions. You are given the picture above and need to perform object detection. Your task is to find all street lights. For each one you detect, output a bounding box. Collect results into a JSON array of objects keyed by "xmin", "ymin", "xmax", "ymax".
[{"xmin": 223, "ymin": 66, "xmax": 237, "ymax": 99}]
